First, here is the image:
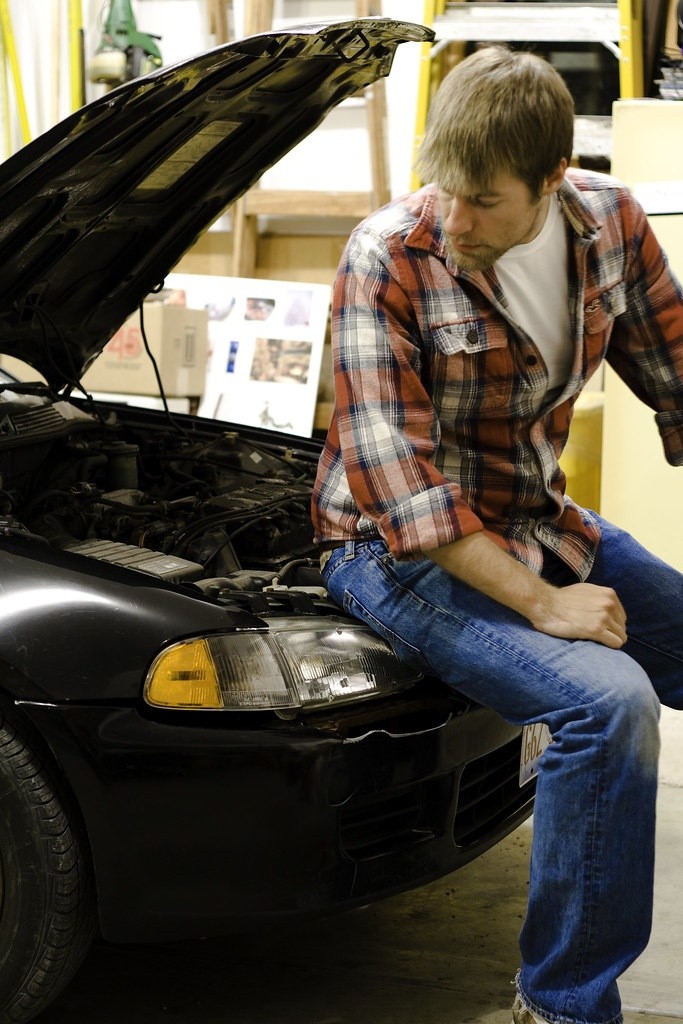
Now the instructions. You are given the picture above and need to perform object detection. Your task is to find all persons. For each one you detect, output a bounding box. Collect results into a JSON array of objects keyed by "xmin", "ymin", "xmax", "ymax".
[{"xmin": 312, "ymin": 45, "xmax": 683, "ymax": 1024}]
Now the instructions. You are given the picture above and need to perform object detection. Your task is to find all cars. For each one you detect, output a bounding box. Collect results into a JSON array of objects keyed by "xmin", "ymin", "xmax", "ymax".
[{"xmin": 0, "ymin": 14, "xmax": 555, "ymax": 1024}]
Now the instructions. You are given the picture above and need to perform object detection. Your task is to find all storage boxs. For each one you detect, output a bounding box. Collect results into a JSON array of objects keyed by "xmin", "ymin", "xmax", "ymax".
[{"xmin": 80, "ymin": 297, "xmax": 211, "ymax": 399}]
